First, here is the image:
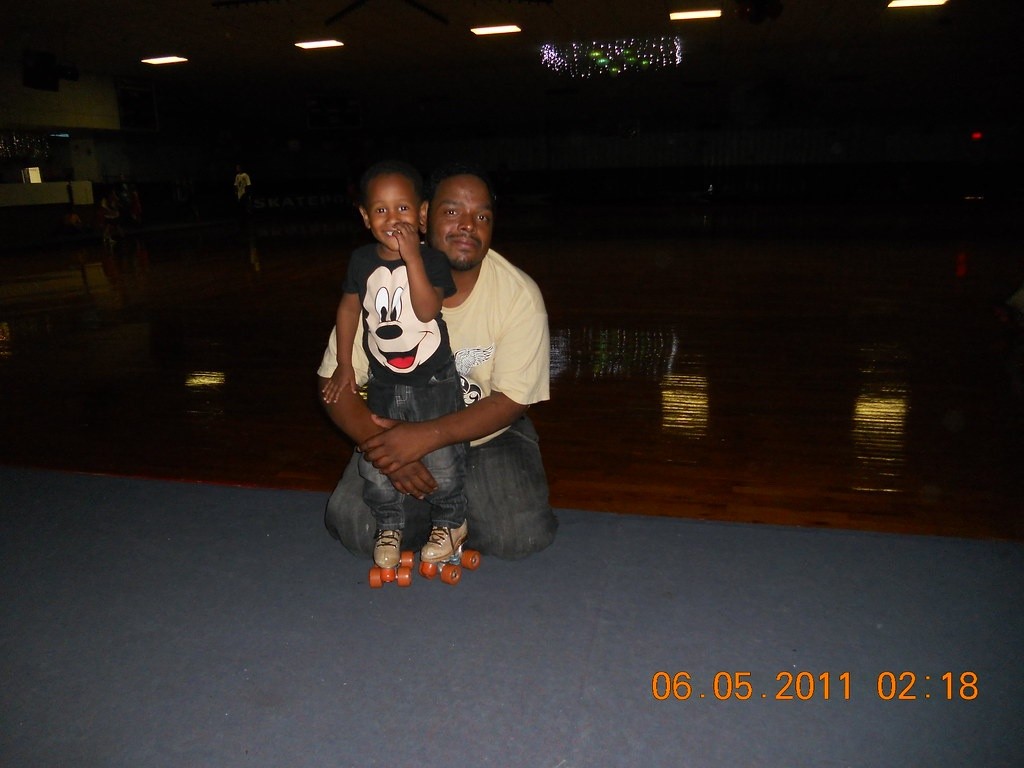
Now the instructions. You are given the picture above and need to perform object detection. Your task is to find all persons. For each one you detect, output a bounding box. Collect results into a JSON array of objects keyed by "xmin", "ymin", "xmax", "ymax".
[
  {"xmin": 322, "ymin": 166, "xmax": 470, "ymax": 562},
  {"xmin": 325, "ymin": 175, "xmax": 559, "ymax": 561}
]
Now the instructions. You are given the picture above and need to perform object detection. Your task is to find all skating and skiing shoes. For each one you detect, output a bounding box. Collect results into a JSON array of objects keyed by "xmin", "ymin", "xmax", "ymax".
[
  {"xmin": 368, "ymin": 528, "xmax": 414, "ymax": 588},
  {"xmin": 418, "ymin": 516, "xmax": 481, "ymax": 585}
]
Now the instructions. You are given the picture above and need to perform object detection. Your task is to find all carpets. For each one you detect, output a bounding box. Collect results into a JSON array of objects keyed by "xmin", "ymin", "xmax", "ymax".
[{"xmin": 0, "ymin": 469, "xmax": 1024, "ymax": 768}]
